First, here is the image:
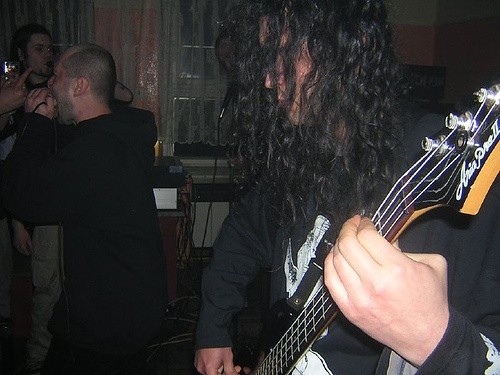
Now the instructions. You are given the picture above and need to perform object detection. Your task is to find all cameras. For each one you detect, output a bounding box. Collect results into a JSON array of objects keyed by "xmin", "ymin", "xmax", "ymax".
[{"xmin": 3, "ymin": 61, "xmax": 20, "ymax": 88}]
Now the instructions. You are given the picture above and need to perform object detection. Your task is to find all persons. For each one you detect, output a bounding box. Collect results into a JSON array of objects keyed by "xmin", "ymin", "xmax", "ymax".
[
  {"xmin": 0, "ymin": 44, "xmax": 176, "ymax": 374},
  {"xmin": 194, "ymin": 0, "xmax": 500, "ymax": 375},
  {"xmin": 0, "ymin": 22, "xmax": 77, "ymax": 374}
]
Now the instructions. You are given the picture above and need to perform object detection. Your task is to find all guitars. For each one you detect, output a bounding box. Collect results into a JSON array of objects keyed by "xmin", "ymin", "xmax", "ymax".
[{"xmin": 154, "ymin": 81, "xmax": 500, "ymax": 374}]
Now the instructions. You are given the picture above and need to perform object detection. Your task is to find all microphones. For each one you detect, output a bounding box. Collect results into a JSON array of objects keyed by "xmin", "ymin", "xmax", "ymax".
[{"xmin": 219, "ymin": 85, "xmax": 234, "ymax": 118}]
